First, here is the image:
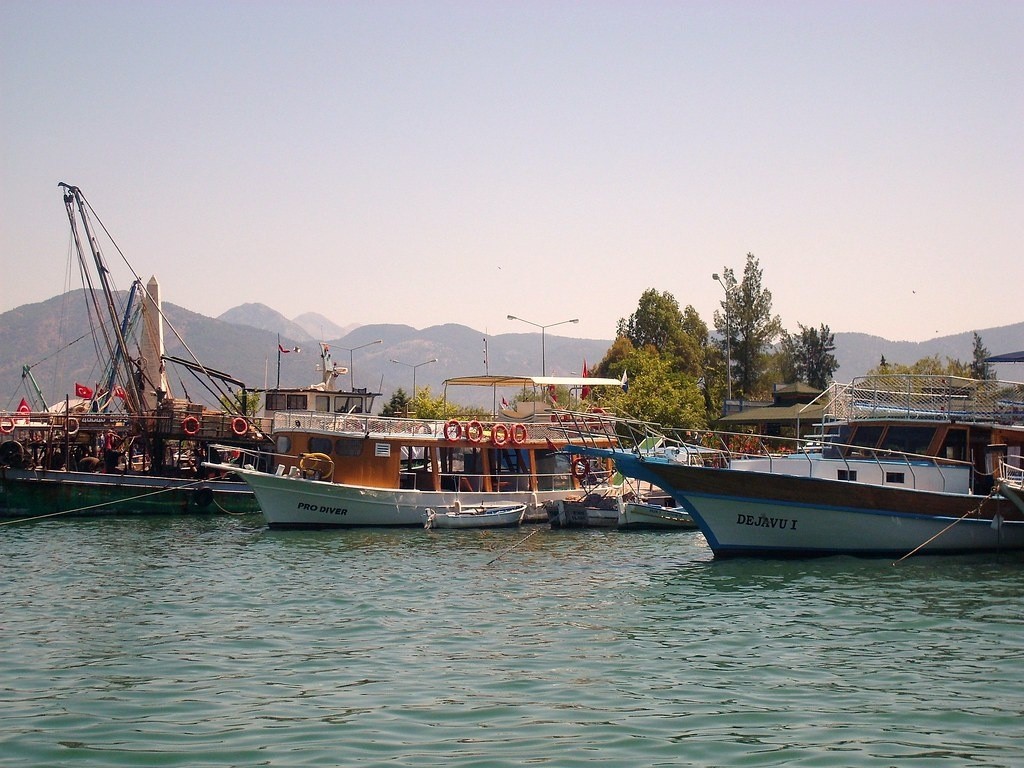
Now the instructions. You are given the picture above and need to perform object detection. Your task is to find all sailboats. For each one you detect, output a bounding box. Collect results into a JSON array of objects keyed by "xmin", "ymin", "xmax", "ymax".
[{"xmin": 0, "ymin": 182, "xmax": 383, "ymax": 518}]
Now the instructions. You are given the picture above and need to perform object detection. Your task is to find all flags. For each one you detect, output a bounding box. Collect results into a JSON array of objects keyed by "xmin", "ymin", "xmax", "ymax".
[
  {"xmin": 112, "ymin": 384, "xmax": 126, "ymax": 400},
  {"xmin": 547, "ymin": 367, "xmax": 558, "ymax": 404},
  {"xmin": 13, "ymin": 397, "xmax": 31, "ymax": 426},
  {"xmin": 94, "ymin": 381, "xmax": 105, "ymax": 399},
  {"xmin": 75, "ymin": 381, "xmax": 92, "ymax": 399},
  {"xmin": 502, "ymin": 398, "xmax": 508, "ymax": 408},
  {"xmin": 277, "ymin": 333, "xmax": 290, "ymax": 354},
  {"xmin": 620, "ymin": 368, "xmax": 628, "ymax": 393},
  {"xmin": 581, "ymin": 357, "xmax": 589, "ymax": 400}
]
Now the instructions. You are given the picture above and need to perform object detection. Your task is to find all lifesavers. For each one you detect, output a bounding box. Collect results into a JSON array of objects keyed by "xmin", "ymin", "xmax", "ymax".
[
  {"xmin": 491, "ymin": 424, "xmax": 509, "ymax": 447},
  {"xmin": 589, "ymin": 409, "xmax": 608, "ymax": 430},
  {"xmin": 510, "ymin": 424, "xmax": 527, "ymax": 446},
  {"xmin": 231, "ymin": 418, "xmax": 248, "ymax": 436},
  {"xmin": 31, "ymin": 430, "xmax": 45, "ymax": 442},
  {"xmin": 571, "ymin": 458, "xmax": 590, "ymax": 479},
  {"xmin": 465, "ymin": 420, "xmax": 484, "ymax": 444},
  {"xmin": 551, "ymin": 407, "xmax": 570, "ymax": 422},
  {"xmin": 0, "ymin": 417, "xmax": 15, "ymax": 433},
  {"xmin": 183, "ymin": 417, "xmax": 200, "ymax": 437},
  {"xmin": 63, "ymin": 417, "xmax": 80, "ymax": 435},
  {"xmin": 444, "ymin": 420, "xmax": 463, "ymax": 443}
]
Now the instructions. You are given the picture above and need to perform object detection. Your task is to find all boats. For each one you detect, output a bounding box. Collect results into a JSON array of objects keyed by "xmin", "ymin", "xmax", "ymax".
[
  {"xmin": 426, "ymin": 502, "xmax": 528, "ymax": 529},
  {"xmin": 616, "ymin": 496, "xmax": 699, "ymax": 532},
  {"xmin": 541, "ymin": 492, "xmax": 682, "ymax": 529},
  {"xmin": 201, "ymin": 375, "xmax": 664, "ymax": 532},
  {"xmin": 544, "ymin": 352, "xmax": 1024, "ymax": 561}
]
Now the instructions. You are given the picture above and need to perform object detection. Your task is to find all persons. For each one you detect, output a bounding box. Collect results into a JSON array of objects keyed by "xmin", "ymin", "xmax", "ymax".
[
  {"xmin": 89, "ymin": 394, "xmax": 101, "ymax": 414},
  {"xmin": 691, "ymin": 432, "xmax": 703, "ymax": 447},
  {"xmin": 27, "ymin": 430, "xmax": 130, "ymax": 475}
]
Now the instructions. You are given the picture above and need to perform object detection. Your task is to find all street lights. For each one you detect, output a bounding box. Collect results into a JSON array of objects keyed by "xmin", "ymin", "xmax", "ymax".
[
  {"xmin": 507, "ymin": 315, "xmax": 579, "ymax": 403},
  {"xmin": 394, "ymin": 359, "xmax": 438, "ymax": 400},
  {"xmin": 319, "ymin": 340, "xmax": 382, "ymax": 406},
  {"xmin": 712, "ymin": 273, "xmax": 741, "ymax": 399}
]
